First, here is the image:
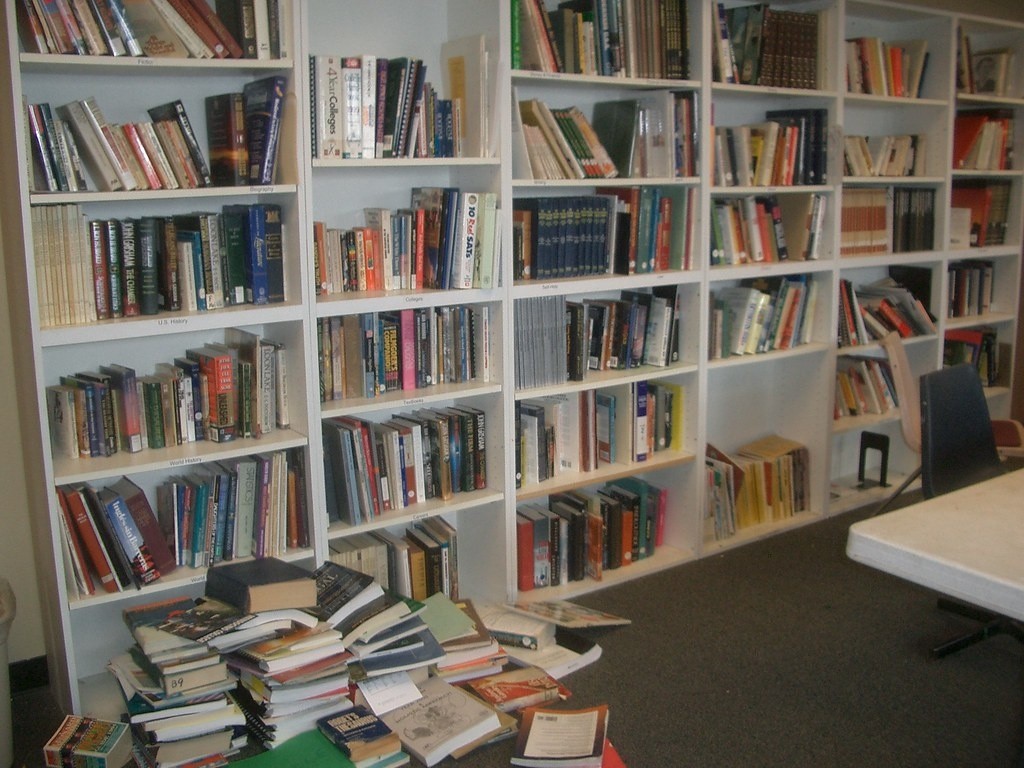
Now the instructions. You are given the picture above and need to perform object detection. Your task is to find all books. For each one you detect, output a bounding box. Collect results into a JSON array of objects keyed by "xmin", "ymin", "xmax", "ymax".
[
  {"xmin": 308, "ymin": 33, "xmax": 494, "ymax": 159},
  {"xmin": 705, "ymin": 435, "xmax": 810, "ymax": 542},
  {"xmin": 513, "ymin": 186, "xmax": 697, "ymax": 282},
  {"xmin": 515, "ymin": 379, "xmax": 683, "ymax": 489},
  {"xmin": 710, "ymin": 102, "xmax": 1015, "ymax": 187},
  {"xmin": 42, "ymin": 447, "xmax": 630, "ymax": 767},
  {"xmin": 833, "ymin": 327, "xmax": 998, "ymax": 420},
  {"xmin": 512, "ymin": 86, "xmax": 700, "ymax": 180},
  {"xmin": 711, "ymin": 181, "xmax": 1013, "ymax": 266},
  {"xmin": 313, "ymin": 187, "xmax": 501, "ymax": 297},
  {"xmin": 21, "ymin": 75, "xmax": 288, "ymax": 192},
  {"xmin": 709, "ymin": 259, "xmax": 995, "ymax": 360},
  {"xmin": 711, "ymin": 0, "xmax": 1016, "ymax": 98},
  {"xmin": 46, "ymin": 327, "xmax": 291, "ymax": 459},
  {"xmin": 316, "ymin": 305, "xmax": 489, "ymax": 400},
  {"xmin": 322, "ymin": 404, "xmax": 487, "ymax": 526},
  {"xmin": 32, "ymin": 204, "xmax": 289, "ymax": 328},
  {"xmin": 517, "ymin": 476, "xmax": 666, "ymax": 592},
  {"xmin": 510, "ymin": 0, "xmax": 692, "ymax": 80},
  {"xmin": 513, "ymin": 284, "xmax": 682, "ymax": 391},
  {"xmin": 13, "ymin": 0, "xmax": 293, "ymax": 61}
]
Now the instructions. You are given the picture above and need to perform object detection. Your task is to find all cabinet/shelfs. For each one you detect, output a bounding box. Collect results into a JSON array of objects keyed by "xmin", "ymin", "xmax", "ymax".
[{"xmin": 0, "ymin": 0, "xmax": 1024, "ymax": 721}]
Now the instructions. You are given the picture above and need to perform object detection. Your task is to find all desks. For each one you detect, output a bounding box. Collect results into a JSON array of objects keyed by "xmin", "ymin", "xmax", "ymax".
[{"xmin": 845, "ymin": 468, "xmax": 1024, "ymax": 622}]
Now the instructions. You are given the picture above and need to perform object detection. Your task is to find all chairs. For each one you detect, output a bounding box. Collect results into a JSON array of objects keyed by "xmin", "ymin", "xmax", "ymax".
[{"xmin": 919, "ymin": 364, "xmax": 1024, "ymax": 659}]
